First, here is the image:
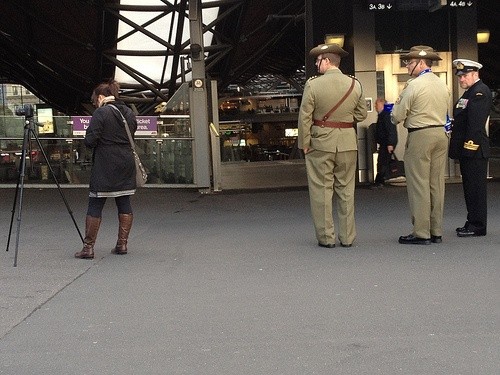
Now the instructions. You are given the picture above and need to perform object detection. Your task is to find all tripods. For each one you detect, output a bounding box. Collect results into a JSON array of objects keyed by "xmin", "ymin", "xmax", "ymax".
[{"xmin": 6, "ymin": 119, "xmax": 84, "ymax": 267}]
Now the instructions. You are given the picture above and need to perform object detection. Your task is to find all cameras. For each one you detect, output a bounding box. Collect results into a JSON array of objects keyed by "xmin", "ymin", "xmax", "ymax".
[{"xmin": 14, "ymin": 104, "xmax": 34, "ymax": 118}]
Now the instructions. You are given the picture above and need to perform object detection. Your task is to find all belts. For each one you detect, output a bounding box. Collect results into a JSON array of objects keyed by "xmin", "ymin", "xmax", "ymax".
[{"xmin": 408, "ymin": 125, "xmax": 444, "ymax": 132}]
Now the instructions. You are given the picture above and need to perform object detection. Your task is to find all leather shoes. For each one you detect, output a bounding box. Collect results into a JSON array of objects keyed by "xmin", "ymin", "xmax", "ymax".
[
  {"xmin": 431, "ymin": 235, "xmax": 442, "ymax": 243},
  {"xmin": 456, "ymin": 227, "xmax": 466, "ymax": 231},
  {"xmin": 399, "ymin": 234, "xmax": 431, "ymax": 244},
  {"xmin": 458, "ymin": 230, "xmax": 475, "ymax": 236}
]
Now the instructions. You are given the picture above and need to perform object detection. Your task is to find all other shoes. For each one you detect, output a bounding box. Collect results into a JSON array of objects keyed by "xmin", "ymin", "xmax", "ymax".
[
  {"xmin": 318, "ymin": 243, "xmax": 335, "ymax": 247},
  {"xmin": 340, "ymin": 242, "xmax": 352, "ymax": 247}
]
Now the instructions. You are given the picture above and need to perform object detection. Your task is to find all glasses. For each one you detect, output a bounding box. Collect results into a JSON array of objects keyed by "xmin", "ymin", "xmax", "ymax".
[{"xmin": 406, "ymin": 61, "xmax": 417, "ymax": 65}]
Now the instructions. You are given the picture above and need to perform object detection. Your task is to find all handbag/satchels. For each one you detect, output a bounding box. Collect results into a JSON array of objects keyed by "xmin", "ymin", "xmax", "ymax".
[
  {"xmin": 384, "ymin": 151, "xmax": 406, "ymax": 176},
  {"xmin": 133, "ymin": 151, "xmax": 148, "ymax": 188}
]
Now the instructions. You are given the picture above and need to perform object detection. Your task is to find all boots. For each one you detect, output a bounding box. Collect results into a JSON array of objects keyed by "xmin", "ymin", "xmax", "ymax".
[
  {"xmin": 75, "ymin": 216, "xmax": 101, "ymax": 257},
  {"xmin": 111, "ymin": 213, "xmax": 133, "ymax": 253}
]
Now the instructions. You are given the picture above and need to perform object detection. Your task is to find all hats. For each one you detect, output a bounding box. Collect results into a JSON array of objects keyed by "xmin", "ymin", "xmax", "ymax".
[
  {"xmin": 400, "ymin": 45, "xmax": 442, "ymax": 60},
  {"xmin": 309, "ymin": 43, "xmax": 349, "ymax": 58},
  {"xmin": 452, "ymin": 59, "xmax": 483, "ymax": 75}
]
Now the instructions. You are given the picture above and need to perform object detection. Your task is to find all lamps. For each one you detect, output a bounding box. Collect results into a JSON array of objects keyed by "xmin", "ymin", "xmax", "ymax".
[
  {"xmin": 324, "ymin": 33, "xmax": 345, "ymax": 48},
  {"xmin": 476, "ymin": 28, "xmax": 490, "ymax": 43}
]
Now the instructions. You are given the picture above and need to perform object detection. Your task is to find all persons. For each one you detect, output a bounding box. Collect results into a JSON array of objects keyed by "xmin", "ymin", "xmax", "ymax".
[
  {"xmin": 448, "ymin": 58, "xmax": 491, "ymax": 237},
  {"xmin": 371, "ymin": 98, "xmax": 398, "ymax": 189},
  {"xmin": 74, "ymin": 81, "xmax": 137, "ymax": 259},
  {"xmin": 297, "ymin": 43, "xmax": 368, "ymax": 248},
  {"xmin": 390, "ymin": 46, "xmax": 451, "ymax": 245}
]
